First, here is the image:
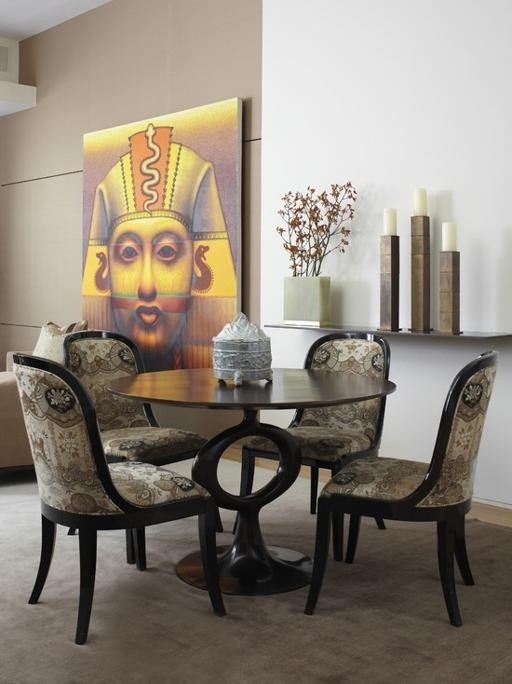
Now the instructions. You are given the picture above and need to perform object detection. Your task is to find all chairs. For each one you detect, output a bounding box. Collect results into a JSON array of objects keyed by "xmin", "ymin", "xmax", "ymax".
[
  {"xmin": 303, "ymin": 347, "xmax": 497, "ymax": 630},
  {"xmin": 232, "ymin": 332, "xmax": 395, "ymax": 565},
  {"xmin": 63, "ymin": 331, "xmax": 226, "ymax": 567},
  {"xmin": 13, "ymin": 350, "xmax": 227, "ymax": 641}
]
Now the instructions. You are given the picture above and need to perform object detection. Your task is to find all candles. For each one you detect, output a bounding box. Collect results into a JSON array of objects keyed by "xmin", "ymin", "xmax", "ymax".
[
  {"xmin": 411, "ymin": 188, "xmax": 428, "ymax": 216},
  {"xmin": 442, "ymin": 223, "xmax": 457, "ymax": 252},
  {"xmin": 383, "ymin": 208, "xmax": 398, "ymax": 236}
]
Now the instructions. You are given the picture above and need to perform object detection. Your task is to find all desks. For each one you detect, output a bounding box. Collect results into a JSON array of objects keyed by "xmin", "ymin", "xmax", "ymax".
[{"xmin": 105, "ymin": 360, "xmax": 400, "ymax": 603}]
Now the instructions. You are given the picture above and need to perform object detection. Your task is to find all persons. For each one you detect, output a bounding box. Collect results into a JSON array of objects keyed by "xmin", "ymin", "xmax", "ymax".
[{"xmin": 106, "ymin": 212, "xmax": 194, "ymax": 359}]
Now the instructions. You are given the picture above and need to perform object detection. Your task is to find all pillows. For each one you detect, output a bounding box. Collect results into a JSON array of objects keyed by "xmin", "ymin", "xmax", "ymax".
[{"xmin": 31, "ymin": 321, "xmax": 88, "ymax": 366}]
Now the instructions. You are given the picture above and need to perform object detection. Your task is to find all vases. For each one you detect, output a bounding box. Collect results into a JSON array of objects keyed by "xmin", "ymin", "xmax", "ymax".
[{"xmin": 283, "ymin": 276, "xmax": 336, "ymax": 328}]
